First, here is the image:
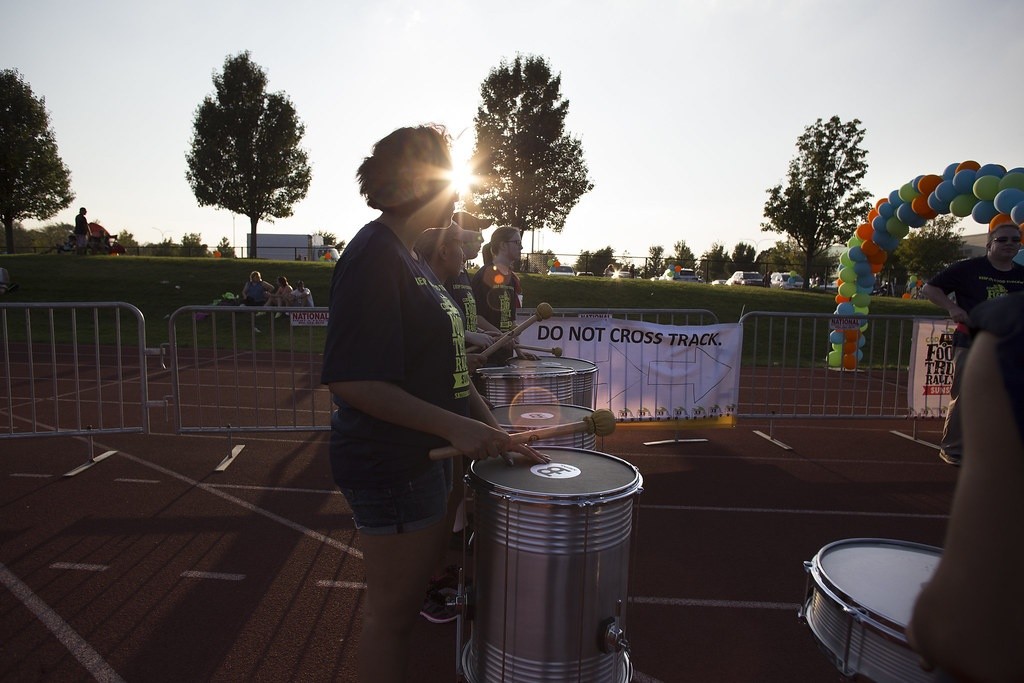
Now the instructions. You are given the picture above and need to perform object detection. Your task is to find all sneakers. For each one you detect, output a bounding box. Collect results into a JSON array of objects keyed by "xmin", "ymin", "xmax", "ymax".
[
  {"xmin": 419, "ymin": 595, "xmax": 457, "ymax": 623},
  {"xmin": 424, "ymin": 574, "xmax": 473, "ymax": 596}
]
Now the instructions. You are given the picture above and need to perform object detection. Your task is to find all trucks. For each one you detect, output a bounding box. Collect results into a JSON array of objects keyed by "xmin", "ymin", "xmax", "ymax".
[{"xmin": 247, "ymin": 233, "xmax": 340, "ymax": 263}]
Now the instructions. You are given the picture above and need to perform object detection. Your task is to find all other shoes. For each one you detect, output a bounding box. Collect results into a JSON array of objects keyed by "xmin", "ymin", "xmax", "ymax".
[{"xmin": 938, "ymin": 449, "xmax": 961, "ymax": 464}]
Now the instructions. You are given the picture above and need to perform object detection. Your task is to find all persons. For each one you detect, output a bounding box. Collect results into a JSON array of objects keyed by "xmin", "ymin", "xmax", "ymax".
[
  {"xmin": 57, "ymin": 207, "xmax": 110, "ymax": 255},
  {"xmin": 905, "ymin": 294, "xmax": 1024, "ymax": 683},
  {"xmin": 319, "ymin": 124, "xmax": 553, "ymax": 683},
  {"xmin": 242, "ymin": 271, "xmax": 315, "ymax": 334},
  {"xmin": 922, "ymin": 213, "xmax": 1024, "ymax": 466},
  {"xmin": 415, "ymin": 211, "xmax": 543, "ymax": 623}
]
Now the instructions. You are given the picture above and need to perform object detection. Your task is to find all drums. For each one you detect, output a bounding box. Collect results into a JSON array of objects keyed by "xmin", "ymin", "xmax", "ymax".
[
  {"xmin": 488, "ymin": 401, "xmax": 598, "ymax": 451},
  {"xmin": 454, "ymin": 444, "xmax": 645, "ymax": 683},
  {"xmin": 796, "ymin": 537, "xmax": 946, "ymax": 683},
  {"xmin": 473, "ymin": 365, "xmax": 578, "ymax": 408},
  {"xmin": 503, "ymin": 355, "xmax": 600, "ymax": 410}
]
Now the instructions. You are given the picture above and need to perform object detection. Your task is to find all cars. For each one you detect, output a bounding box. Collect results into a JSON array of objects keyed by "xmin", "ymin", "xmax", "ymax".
[
  {"xmin": 547, "ymin": 265, "xmax": 576, "ymax": 276},
  {"xmin": 771, "ymin": 272, "xmax": 804, "ymax": 289},
  {"xmin": 731, "ymin": 271, "xmax": 764, "ymax": 285}
]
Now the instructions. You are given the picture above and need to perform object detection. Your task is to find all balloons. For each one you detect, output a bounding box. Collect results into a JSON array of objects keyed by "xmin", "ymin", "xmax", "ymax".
[
  {"xmin": 320, "ymin": 253, "xmax": 332, "ymax": 262},
  {"xmin": 547, "ymin": 260, "xmax": 561, "ymax": 272},
  {"xmin": 908, "ymin": 282, "xmax": 916, "ymax": 288},
  {"xmin": 917, "ymin": 280, "xmax": 922, "ymax": 286},
  {"xmin": 825, "ymin": 161, "xmax": 1024, "ymax": 370},
  {"xmin": 667, "ymin": 265, "xmax": 682, "ymax": 277},
  {"xmin": 902, "ymin": 293, "xmax": 910, "ymax": 298},
  {"xmin": 910, "ymin": 275, "xmax": 917, "ymax": 282},
  {"xmin": 787, "ymin": 271, "xmax": 796, "ymax": 283}
]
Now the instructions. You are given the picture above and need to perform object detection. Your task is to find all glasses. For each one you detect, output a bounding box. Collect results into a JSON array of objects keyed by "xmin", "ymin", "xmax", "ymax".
[
  {"xmin": 992, "ymin": 236, "xmax": 1022, "ymax": 244},
  {"xmin": 505, "ymin": 240, "xmax": 522, "ymax": 245}
]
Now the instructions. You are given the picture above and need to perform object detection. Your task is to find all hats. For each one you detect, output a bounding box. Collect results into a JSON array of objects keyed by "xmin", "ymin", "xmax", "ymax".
[
  {"xmin": 414, "ymin": 220, "xmax": 482, "ymax": 249},
  {"xmin": 451, "ymin": 212, "xmax": 495, "ymax": 231}
]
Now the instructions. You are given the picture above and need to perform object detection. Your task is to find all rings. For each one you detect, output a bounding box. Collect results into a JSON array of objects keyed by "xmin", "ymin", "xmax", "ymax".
[{"xmin": 525, "ymin": 444, "xmax": 527, "ymax": 446}]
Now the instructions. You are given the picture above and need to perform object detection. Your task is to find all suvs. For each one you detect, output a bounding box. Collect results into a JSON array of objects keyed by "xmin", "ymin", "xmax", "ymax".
[{"xmin": 673, "ymin": 268, "xmax": 698, "ymax": 283}]
